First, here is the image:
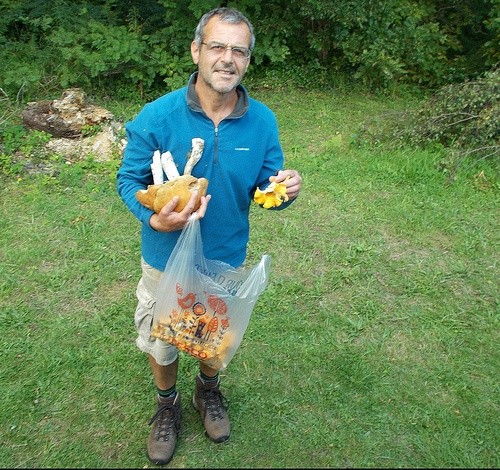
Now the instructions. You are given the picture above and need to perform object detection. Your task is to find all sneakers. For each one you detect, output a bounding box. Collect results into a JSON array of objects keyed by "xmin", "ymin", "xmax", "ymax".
[
  {"xmin": 193, "ymin": 372, "xmax": 231, "ymax": 442},
  {"xmin": 147, "ymin": 392, "xmax": 181, "ymax": 464}
]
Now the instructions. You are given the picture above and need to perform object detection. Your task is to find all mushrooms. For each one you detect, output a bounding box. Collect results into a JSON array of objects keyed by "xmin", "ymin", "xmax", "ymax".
[{"xmin": 134, "ymin": 138, "xmax": 208, "ymax": 214}]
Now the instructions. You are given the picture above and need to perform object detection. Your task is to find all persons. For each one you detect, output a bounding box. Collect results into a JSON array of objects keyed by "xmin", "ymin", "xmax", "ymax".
[{"xmin": 115, "ymin": 6, "xmax": 304, "ymax": 466}]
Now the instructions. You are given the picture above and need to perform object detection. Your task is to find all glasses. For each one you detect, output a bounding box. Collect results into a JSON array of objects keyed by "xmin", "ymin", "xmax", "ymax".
[{"xmin": 200, "ymin": 40, "xmax": 253, "ymax": 57}]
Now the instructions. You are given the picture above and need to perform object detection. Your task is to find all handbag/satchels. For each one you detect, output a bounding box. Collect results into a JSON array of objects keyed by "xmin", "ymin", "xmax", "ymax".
[{"xmin": 148, "ymin": 212, "xmax": 271, "ymax": 371}]
